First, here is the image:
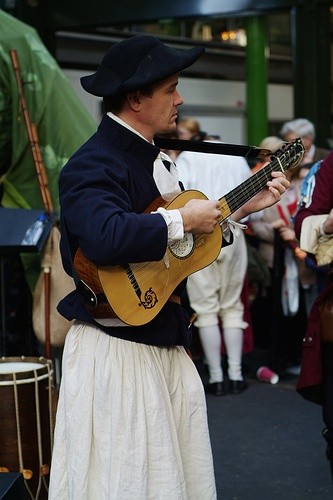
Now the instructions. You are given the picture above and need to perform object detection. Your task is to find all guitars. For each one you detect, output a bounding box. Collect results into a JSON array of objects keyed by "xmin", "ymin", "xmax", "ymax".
[{"xmin": 73, "ymin": 137, "xmax": 307, "ymax": 327}]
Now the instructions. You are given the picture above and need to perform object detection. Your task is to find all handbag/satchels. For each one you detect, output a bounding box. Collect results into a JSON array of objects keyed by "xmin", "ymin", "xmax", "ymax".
[{"xmin": 33, "ymin": 225, "xmax": 76, "ymax": 348}]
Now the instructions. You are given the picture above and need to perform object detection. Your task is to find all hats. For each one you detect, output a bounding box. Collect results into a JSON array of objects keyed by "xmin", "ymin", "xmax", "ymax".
[{"xmin": 80, "ymin": 33, "xmax": 207, "ymax": 100}]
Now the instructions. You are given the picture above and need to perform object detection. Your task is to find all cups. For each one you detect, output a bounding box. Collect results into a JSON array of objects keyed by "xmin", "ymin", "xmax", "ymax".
[{"xmin": 257, "ymin": 366, "xmax": 279, "ymax": 385}]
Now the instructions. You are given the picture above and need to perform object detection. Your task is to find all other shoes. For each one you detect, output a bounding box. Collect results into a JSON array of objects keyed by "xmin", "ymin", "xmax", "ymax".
[
  {"xmin": 205, "ymin": 379, "xmax": 225, "ymax": 395},
  {"xmin": 229, "ymin": 377, "xmax": 249, "ymax": 394}
]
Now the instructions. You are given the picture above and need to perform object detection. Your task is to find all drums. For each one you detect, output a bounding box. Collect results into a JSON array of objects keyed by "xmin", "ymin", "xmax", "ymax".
[{"xmin": 0, "ymin": 357, "xmax": 54, "ymax": 479}]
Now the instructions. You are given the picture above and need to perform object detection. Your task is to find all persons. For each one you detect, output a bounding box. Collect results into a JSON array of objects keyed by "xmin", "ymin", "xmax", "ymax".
[
  {"xmin": 159, "ymin": 118, "xmax": 333, "ymax": 466},
  {"xmin": 49, "ymin": 35, "xmax": 291, "ymax": 500}
]
